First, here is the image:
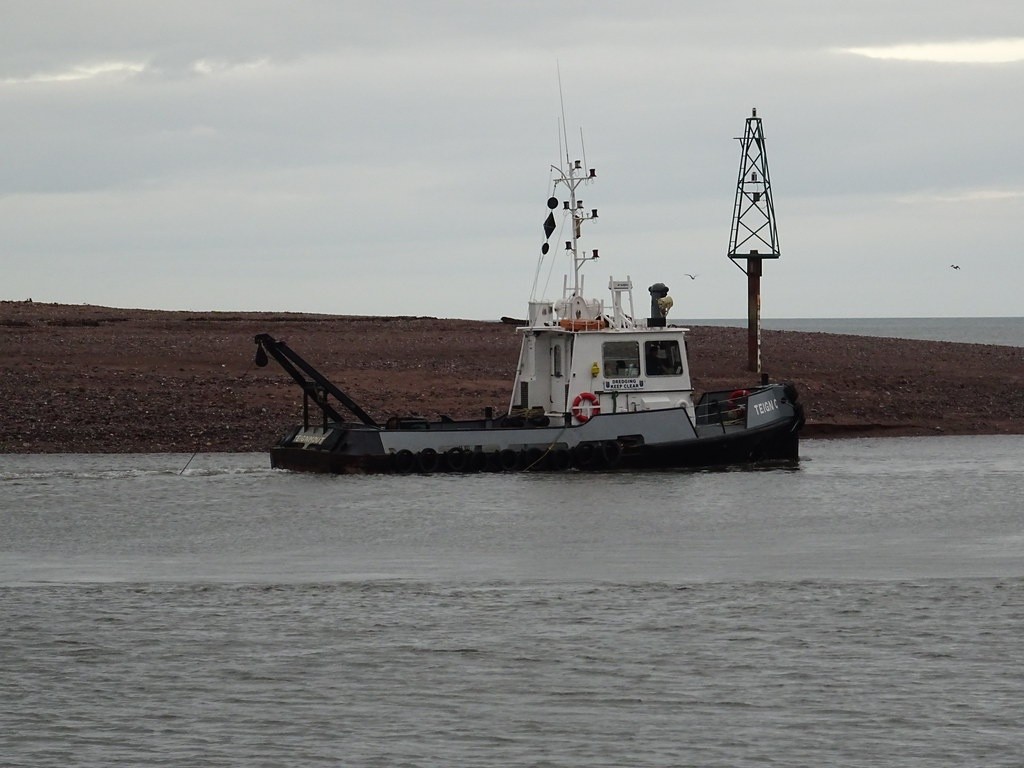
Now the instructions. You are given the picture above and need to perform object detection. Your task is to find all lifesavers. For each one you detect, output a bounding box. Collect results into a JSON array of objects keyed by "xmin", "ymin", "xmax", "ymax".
[
  {"xmin": 576, "ymin": 441, "xmax": 598, "ymax": 468},
  {"xmin": 446, "ymin": 447, "xmax": 468, "ymax": 471},
  {"xmin": 470, "ymin": 450, "xmax": 489, "ymax": 474},
  {"xmin": 572, "ymin": 392, "xmax": 600, "ymax": 421},
  {"xmin": 602, "ymin": 439, "xmax": 622, "ymax": 466},
  {"xmin": 418, "ymin": 448, "xmax": 439, "ymax": 472},
  {"xmin": 498, "ymin": 449, "xmax": 520, "ymax": 470},
  {"xmin": 726, "ymin": 390, "xmax": 752, "ymax": 414},
  {"xmin": 395, "ymin": 449, "xmax": 415, "ymax": 472},
  {"xmin": 559, "ymin": 318, "xmax": 606, "ymax": 331}
]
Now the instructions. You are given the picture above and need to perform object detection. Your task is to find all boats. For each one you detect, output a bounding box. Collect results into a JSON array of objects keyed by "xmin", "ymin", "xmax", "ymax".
[
  {"xmin": 560, "ymin": 319, "xmax": 605, "ymax": 330},
  {"xmin": 252, "ymin": 57, "xmax": 805, "ymax": 476}
]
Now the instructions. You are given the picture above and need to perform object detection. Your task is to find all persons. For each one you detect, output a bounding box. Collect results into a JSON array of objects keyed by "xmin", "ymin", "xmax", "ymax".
[{"xmin": 648, "ymin": 346, "xmax": 662, "ymax": 364}]
